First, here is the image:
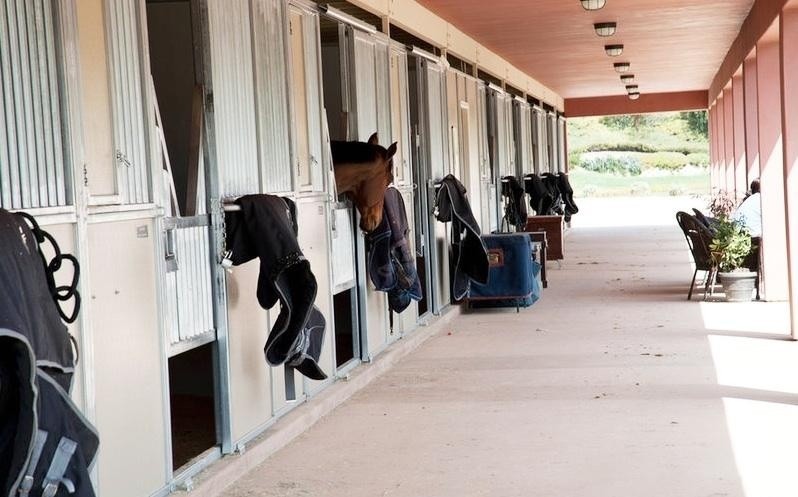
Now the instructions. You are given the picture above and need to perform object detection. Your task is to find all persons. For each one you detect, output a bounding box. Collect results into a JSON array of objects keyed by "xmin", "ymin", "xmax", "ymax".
[{"xmin": 733, "ymin": 176, "xmax": 761, "ymax": 238}]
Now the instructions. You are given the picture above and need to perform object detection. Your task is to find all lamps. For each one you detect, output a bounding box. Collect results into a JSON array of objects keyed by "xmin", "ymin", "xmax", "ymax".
[{"xmin": 580, "ymin": 1, "xmax": 642, "ymax": 101}]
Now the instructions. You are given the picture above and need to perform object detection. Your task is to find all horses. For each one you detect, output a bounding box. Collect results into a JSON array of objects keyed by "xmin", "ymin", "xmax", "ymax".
[{"xmin": 325, "ymin": 131, "xmax": 400, "ymax": 236}]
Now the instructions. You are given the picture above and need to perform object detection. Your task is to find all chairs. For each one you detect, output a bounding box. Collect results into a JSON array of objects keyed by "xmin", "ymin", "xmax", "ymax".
[{"xmin": 675, "ymin": 207, "xmax": 765, "ymax": 303}]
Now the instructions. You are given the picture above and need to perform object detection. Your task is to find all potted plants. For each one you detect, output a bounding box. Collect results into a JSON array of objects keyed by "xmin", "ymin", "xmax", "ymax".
[{"xmin": 704, "ymin": 185, "xmax": 760, "ymax": 302}]
